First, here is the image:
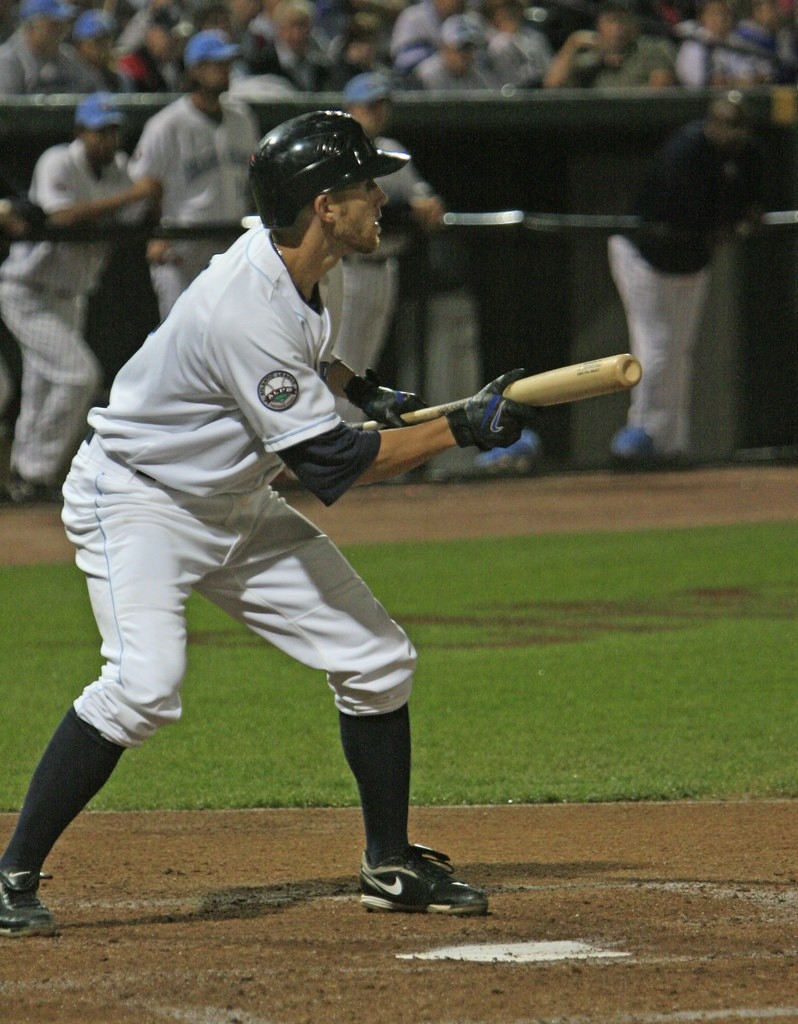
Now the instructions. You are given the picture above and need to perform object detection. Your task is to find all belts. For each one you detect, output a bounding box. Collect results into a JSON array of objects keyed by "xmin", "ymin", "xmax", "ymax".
[{"xmin": 85, "ymin": 428, "xmax": 155, "ymax": 480}]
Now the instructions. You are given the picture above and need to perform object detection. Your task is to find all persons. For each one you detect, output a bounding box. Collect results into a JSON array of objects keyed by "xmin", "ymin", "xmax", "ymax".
[
  {"xmin": 0, "ymin": 112, "xmax": 548, "ymax": 939},
  {"xmin": 0, "ymin": 0, "xmax": 798, "ymax": 95},
  {"xmin": 1, "ymin": 28, "xmax": 445, "ymax": 507},
  {"xmin": 605, "ymin": 84, "xmax": 775, "ymax": 471}
]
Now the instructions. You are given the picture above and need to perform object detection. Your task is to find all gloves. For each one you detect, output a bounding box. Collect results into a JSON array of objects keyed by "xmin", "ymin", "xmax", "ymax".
[
  {"xmin": 343, "ymin": 367, "xmax": 433, "ymax": 428},
  {"xmin": 446, "ymin": 367, "xmax": 541, "ymax": 453}
]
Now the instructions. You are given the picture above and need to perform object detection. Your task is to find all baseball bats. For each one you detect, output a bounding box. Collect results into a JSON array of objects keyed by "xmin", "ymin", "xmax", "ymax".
[{"xmin": 342, "ymin": 353, "xmax": 642, "ymax": 432}]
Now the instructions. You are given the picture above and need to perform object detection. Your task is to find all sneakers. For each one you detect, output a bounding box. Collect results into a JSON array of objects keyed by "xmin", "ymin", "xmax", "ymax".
[
  {"xmin": 362, "ymin": 843, "xmax": 489, "ymax": 915},
  {"xmin": 0, "ymin": 868, "xmax": 62, "ymax": 938}
]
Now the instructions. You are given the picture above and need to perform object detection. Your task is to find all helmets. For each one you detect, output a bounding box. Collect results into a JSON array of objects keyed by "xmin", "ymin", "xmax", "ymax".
[{"xmin": 248, "ymin": 110, "xmax": 412, "ymax": 230}]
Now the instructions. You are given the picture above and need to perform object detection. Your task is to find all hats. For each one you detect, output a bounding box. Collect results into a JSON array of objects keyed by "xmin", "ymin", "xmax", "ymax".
[
  {"xmin": 74, "ymin": 90, "xmax": 132, "ymax": 129},
  {"xmin": 343, "ymin": 71, "xmax": 394, "ymax": 105},
  {"xmin": 72, "ymin": 8, "xmax": 125, "ymax": 42},
  {"xmin": 442, "ymin": 14, "xmax": 484, "ymax": 50},
  {"xmin": 19, "ymin": 0, "xmax": 81, "ymax": 22},
  {"xmin": 183, "ymin": 28, "xmax": 246, "ymax": 66}
]
